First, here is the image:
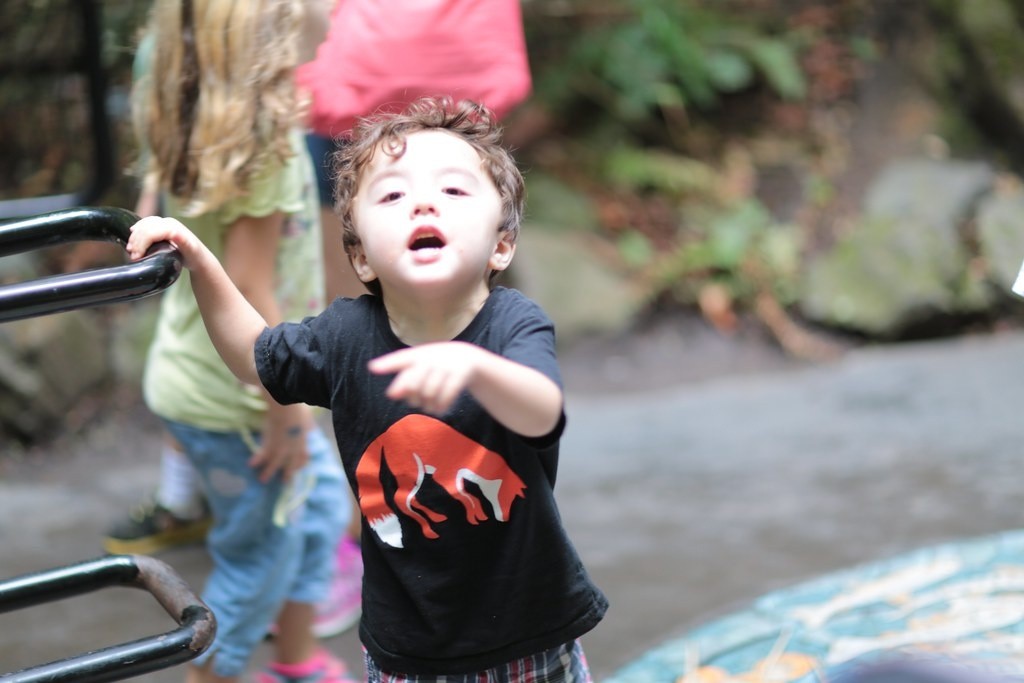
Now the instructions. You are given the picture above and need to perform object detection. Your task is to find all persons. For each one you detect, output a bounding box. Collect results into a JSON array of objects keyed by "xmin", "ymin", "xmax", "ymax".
[
  {"xmin": 130, "ymin": 0, "xmax": 353, "ymax": 683},
  {"xmin": 125, "ymin": 94, "xmax": 608, "ymax": 683},
  {"xmin": 99, "ymin": 0, "xmax": 532, "ymax": 555}
]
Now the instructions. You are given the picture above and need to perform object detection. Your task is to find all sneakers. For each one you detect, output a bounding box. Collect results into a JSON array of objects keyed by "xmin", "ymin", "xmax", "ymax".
[{"xmin": 103, "ymin": 498, "xmax": 209, "ymax": 556}]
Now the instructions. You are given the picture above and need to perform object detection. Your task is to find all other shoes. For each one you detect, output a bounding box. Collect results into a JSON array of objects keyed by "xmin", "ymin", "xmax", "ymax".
[
  {"xmin": 257, "ymin": 649, "xmax": 358, "ymax": 683},
  {"xmin": 314, "ymin": 540, "xmax": 366, "ymax": 639}
]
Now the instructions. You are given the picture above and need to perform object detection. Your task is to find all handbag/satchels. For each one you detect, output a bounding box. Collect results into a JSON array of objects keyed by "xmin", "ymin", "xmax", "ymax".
[{"xmin": 290, "ymin": 1, "xmax": 530, "ymax": 141}]
